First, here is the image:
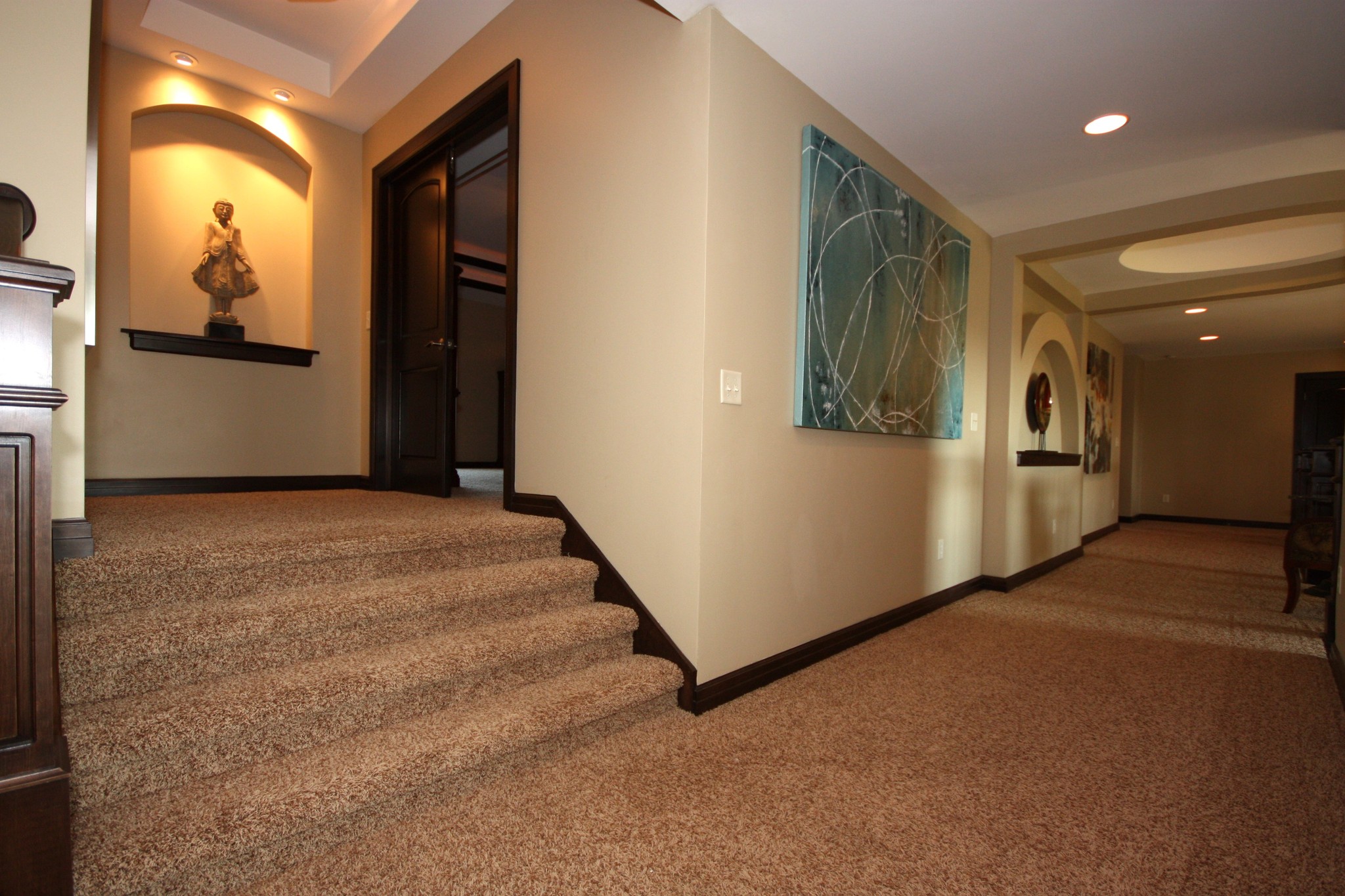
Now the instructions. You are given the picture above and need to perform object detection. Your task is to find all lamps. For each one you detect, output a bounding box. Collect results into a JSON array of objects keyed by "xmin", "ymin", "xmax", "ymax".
[
  {"xmin": 170, "ymin": 51, "xmax": 198, "ymax": 67},
  {"xmin": 270, "ymin": 88, "xmax": 296, "ymax": 102}
]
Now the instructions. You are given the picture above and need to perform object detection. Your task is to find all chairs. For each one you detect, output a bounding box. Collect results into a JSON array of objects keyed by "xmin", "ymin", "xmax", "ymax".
[{"xmin": 1278, "ymin": 446, "xmax": 1338, "ymax": 615}]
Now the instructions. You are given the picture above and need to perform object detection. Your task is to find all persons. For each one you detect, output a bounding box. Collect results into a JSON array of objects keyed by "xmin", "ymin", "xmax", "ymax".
[{"xmin": 191, "ymin": 199, "xmax": 259, "ymax": 324}]
